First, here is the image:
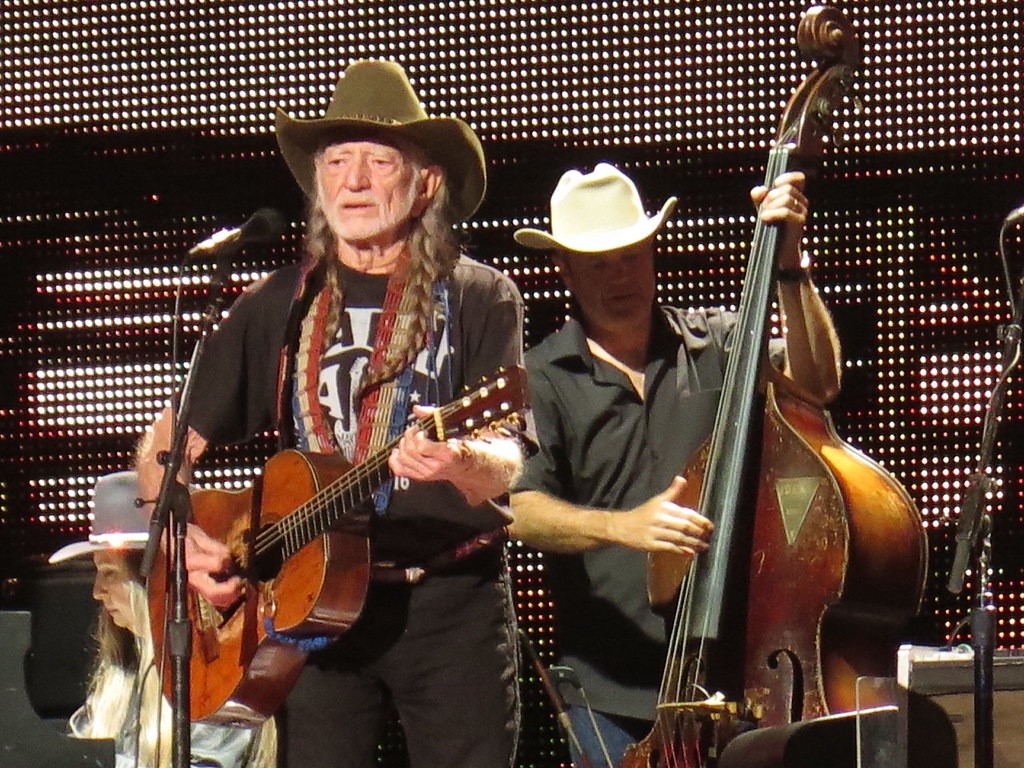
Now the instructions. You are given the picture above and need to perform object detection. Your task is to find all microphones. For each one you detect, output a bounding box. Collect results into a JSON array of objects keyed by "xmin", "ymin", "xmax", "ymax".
[{"xmin": 188, "ymin": 207, "xmax": 282, "ymax": 260}]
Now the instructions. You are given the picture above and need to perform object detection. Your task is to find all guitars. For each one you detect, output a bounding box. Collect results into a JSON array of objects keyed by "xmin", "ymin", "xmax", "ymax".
[{"xmin": 145, "ymin": 359, "xmax": 532, "ymax": 733}]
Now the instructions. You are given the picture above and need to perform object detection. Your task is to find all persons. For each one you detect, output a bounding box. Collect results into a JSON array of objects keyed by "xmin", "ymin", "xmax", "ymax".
[
  {"xmin": 48, "ymin": 471, "xmax": 267, "ymax": 768},
  {"xmin": 138, "ymin": 62, "xmax": 541, "ymax": 768},
  {"xmin": 506, "ymin": 163, "xmax": 841, "ymax": 768}
]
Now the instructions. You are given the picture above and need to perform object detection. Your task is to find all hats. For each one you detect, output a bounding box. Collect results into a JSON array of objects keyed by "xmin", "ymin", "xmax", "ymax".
[
  {"xmin": 274, "ymin": 60, "xmax": 487, "ymax": 225},
  {"xmin": 513, "ymin": 163, "xmax": 677, "ymax": 256},
  {"xmin": 48, "ymin": 471, "xmax": 150, "ymax": 564}
]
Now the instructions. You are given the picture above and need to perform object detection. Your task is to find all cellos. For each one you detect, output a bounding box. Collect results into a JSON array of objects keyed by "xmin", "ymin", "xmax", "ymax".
[{"xmin": 620, "ymin": 5, "xmax": 962, "ymax": 768}]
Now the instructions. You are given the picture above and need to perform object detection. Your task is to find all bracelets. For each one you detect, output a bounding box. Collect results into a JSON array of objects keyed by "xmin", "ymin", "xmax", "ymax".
[{"xmin": 778, "ymin": 250, "xmax": 817, "ymax": 283}]
